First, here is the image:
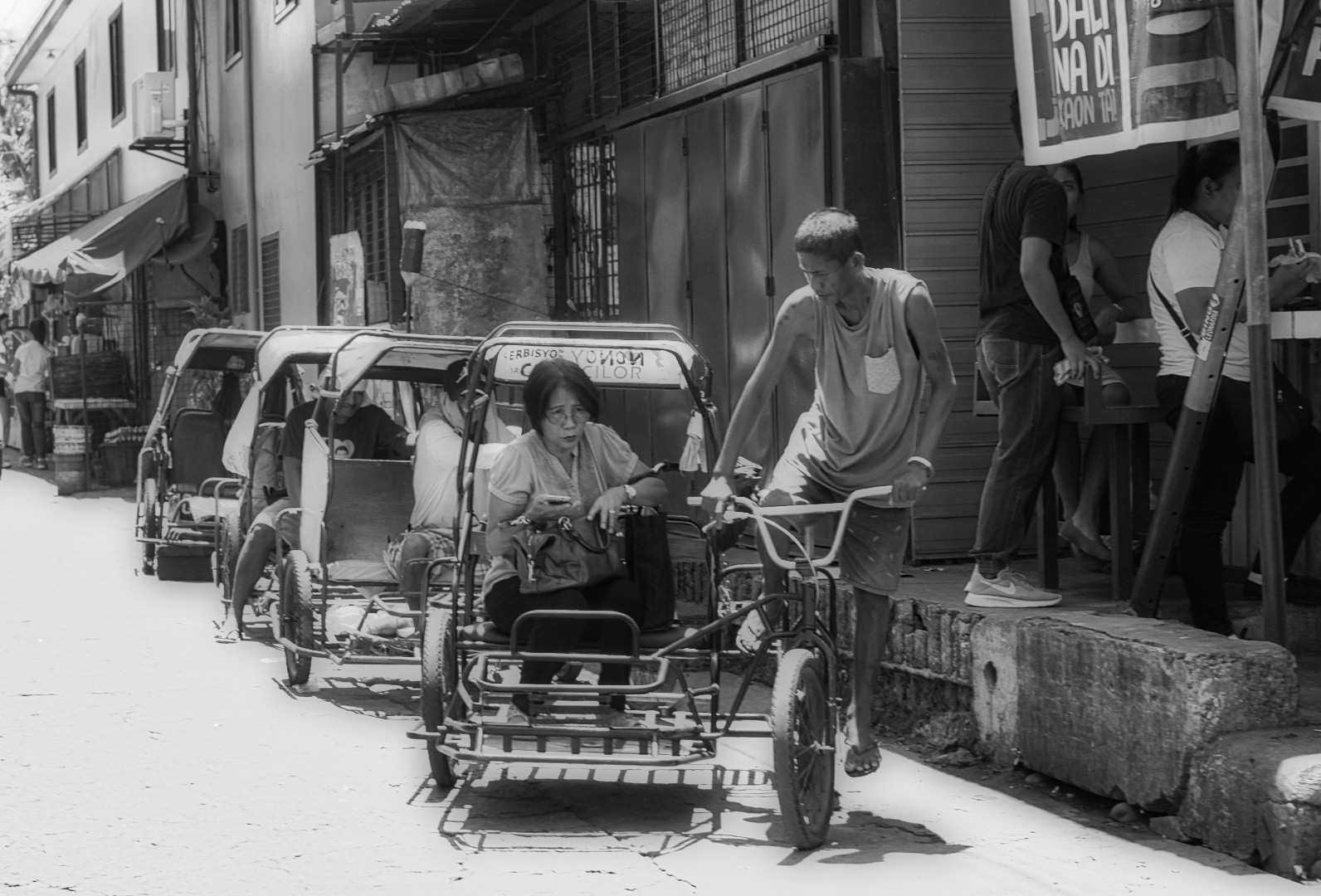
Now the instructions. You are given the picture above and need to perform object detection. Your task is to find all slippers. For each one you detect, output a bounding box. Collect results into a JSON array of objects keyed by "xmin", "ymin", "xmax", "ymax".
[
  {"xmin": 216, "ymin": 627, "xmax": 241, "ymax": 643},
  {"xmin": 843, "ymin": 723, "xmax": 880, "ymax": 777},
  {"xmin": 735, "ymin": 619, "xmax": 766, "ymax": 658}
]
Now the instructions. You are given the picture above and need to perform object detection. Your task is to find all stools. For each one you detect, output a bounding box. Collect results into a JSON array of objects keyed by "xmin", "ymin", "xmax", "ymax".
[{"xmin": 1037, "ymin": 403, "xmax": 1166, "ymax": 601}]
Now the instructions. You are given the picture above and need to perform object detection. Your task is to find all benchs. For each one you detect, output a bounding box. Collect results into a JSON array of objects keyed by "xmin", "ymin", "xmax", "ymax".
[
  {"xmin": 467, "ymin": 515, "xmax": 721, "ymax": 720},
  {"xmin": 324, "ymin": 453, "xmax": 417, "ymax": 591}
]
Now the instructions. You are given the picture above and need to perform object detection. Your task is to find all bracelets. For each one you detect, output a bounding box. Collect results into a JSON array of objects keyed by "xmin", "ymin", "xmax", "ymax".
[{"xmin": 904, "ymin": 456, "xmax": 935, "ymax": 477}]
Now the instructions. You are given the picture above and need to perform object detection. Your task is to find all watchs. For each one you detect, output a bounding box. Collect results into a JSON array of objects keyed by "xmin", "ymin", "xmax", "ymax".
[{"xmin": 621, "ymin": 483, "xmax": 638, "ymax": 506}]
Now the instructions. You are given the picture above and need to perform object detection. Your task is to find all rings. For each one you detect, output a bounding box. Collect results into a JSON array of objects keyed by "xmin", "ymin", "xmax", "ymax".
[{"xmin": 608, "ymin": 510, "xmax": 616, "ymax": 515}]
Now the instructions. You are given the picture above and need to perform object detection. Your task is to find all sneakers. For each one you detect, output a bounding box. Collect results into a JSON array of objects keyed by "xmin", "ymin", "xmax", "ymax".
[
  {"xmin": 964, "ymin": 565, "xmax": 1051, "ymax": 598},
  {"xmin": 963, "ymin": 569, "xmax": 1062, "ymax": 607}
]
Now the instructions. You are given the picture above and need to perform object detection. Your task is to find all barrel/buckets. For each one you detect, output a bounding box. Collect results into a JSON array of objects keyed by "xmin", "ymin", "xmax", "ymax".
[
  {"xmin": 94, "ymin": 444, "xmax": 142, "ymax": 487},
  {"xmin": 53, "ymin": 426, "xmax": 92, "ymax": 494}
]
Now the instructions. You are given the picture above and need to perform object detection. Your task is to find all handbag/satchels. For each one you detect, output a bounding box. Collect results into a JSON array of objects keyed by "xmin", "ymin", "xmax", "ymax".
[
  {"xmin": 1223, "ymin": 369, "xmax": 1317, "ymax": 473},
  {"xmin": 1058, "ymin": 274, "xmax": 1099, "ymax": 346},
  {"xmin": 621, "ymin": 507, "xmax": 676, "ymax": 630},
  {"xmin": 510, "ymin": 508, "xmax": 623, "ymax": 595}
]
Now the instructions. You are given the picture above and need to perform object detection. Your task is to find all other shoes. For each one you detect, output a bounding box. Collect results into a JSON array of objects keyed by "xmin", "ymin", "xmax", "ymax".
[
  {"xmin": 1244, "ymin": 571, "xmax": 1321, "ymax": 607},
  {"xmin": 1101, "ymin": 534, "xmax": 1143, "ymax": 550},
  {"xmin": 1058, "ymin": 519, "xmax": 1111, "ymax": 560},
  {"xmin": 37, "ymin": 458, "xmax": 48, "ymax": 470},
  {"xmin": 2, "ymin": 459, "xmax": 11, "ymax": 469},
  {"xmin": 19, "ymin": 455, "xmax": 32, "ymax": 466}
]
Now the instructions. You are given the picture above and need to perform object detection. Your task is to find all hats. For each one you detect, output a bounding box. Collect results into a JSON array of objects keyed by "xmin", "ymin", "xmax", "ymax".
[{"xmin": 442, "ymin": 358, "xmax": 488, "ymax": 402}]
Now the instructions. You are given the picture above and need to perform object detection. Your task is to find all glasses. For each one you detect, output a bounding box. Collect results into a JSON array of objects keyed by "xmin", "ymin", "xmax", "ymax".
[{"xmin": 544, "ymin": 408, "xmax": 590, "ymax": 425}]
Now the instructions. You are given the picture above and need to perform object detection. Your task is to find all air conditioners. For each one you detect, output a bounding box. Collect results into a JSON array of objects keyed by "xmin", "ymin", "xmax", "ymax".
[{"xmin": 133, "ymin": 70, "xmax": 180, "ymax": 140}]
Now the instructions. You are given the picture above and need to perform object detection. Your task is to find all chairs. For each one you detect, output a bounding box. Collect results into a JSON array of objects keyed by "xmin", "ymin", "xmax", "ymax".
[{"xmin": 166, "ymin": 407, "xmax": 239, "ymax": 498}]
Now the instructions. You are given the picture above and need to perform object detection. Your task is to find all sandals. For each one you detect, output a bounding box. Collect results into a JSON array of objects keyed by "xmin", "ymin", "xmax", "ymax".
[
  {"xmin": 506, "ymin": 703, "xmax": 531, "ymax": 724},
  {"xmin": 594, "ymin": 709, "xmax": 649, "ymax": 729}
]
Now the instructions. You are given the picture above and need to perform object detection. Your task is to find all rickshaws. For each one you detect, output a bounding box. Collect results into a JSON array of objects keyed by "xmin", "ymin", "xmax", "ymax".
[
  {"xmin": 132, "ymin": 318, "xmax": 494, "ymax": 698},
  {"xmin": 410, "ymin": 319, "xmax": 924, "ymax": 852}
]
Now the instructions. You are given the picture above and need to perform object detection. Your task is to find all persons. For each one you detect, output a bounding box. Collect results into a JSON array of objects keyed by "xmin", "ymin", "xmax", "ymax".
[
  {"xmin": 0, "ymin": 312, "xmax": 61, "ymax": 469},
  {"xmin": 962, "ymin": 88, "xmax": 1111, "ymax": 611},
  {"xmin": 397, "ymin": 357, "xmax": 528, "ymax": 641},
  {"xmin": 1038, "ymin": 147, "xmax": 1131, "ymax": 573},
  {"xmin": 480, "ymin": 356, "xmax": 691, "ymax": 731},
  {"xmin": 215, "ymin": 364, "xmax": 418, "ymax": 643},
  {"xmin": 699, "ymin": 205, "xmax": 959, "ymax": 777},
  {"xmin": 1144, "ymin": 135, "xmax": 1321, "ymax": 642}
]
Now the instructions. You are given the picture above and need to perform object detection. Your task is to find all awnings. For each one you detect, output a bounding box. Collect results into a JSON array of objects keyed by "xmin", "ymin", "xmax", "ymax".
[{"xmin": 8, "ymin": 173, "xmax": 199, "ymax": 295}]
